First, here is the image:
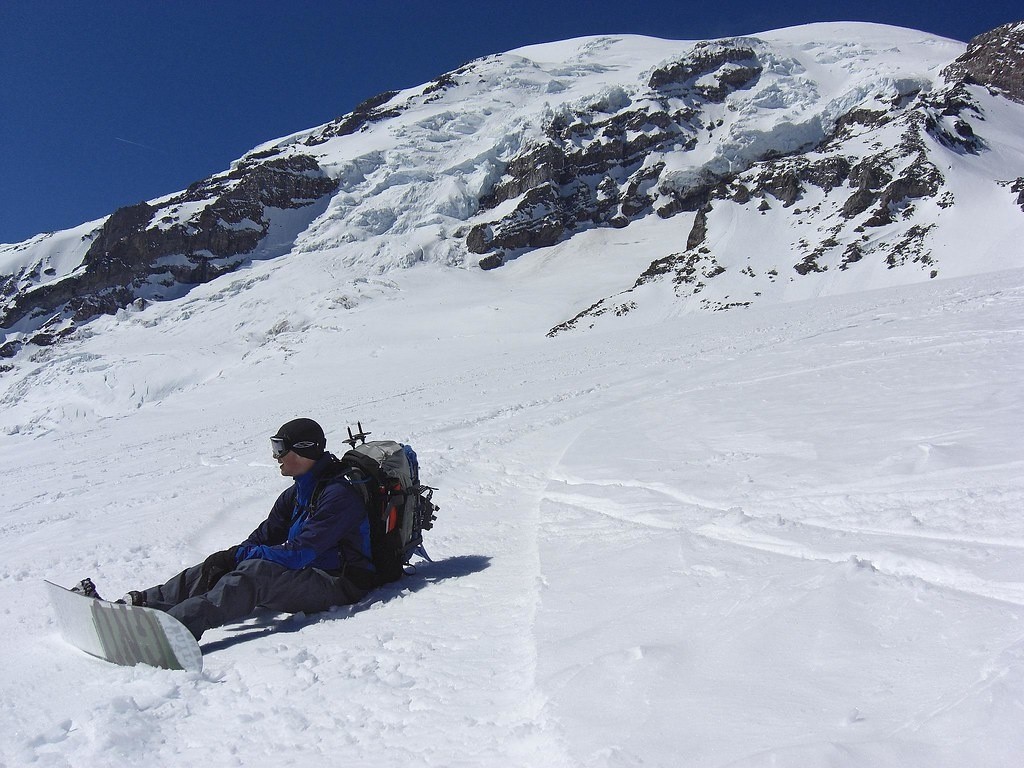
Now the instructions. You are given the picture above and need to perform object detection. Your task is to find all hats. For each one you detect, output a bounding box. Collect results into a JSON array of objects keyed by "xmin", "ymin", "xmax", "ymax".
[{"xmin": 275, "ymin": 418, "xmax": 325, "ymax": 460}]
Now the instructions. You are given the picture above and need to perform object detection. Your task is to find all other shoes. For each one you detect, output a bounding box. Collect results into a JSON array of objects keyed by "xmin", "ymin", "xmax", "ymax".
[{"xmin": 75, "ymin": 580, "xmax": 127, "ymax": 606}]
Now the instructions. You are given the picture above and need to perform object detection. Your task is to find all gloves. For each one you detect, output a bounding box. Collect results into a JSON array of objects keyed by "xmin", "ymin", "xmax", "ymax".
[
  {"xmin": 208, "ymin": 566, "xmax": 231, "ymax": 591},
  {"xmin": 200, "ymin": 545, "xmax": 244, "ymax": 583}
]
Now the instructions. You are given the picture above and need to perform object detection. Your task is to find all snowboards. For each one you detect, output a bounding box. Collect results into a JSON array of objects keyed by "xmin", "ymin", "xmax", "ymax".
[{"xmin": 42, "ymin": 576, "xmax": 204, "ymax": 673}]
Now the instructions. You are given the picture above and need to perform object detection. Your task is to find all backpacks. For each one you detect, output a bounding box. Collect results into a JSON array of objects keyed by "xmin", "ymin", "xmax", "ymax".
[{"xmin": 291, "ymin": 441, "xmax": 440, "ymax": 583}]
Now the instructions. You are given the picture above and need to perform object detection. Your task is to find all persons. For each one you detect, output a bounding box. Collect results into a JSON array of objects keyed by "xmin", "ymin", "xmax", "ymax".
[{"xmin": 70, "ymin": 419, "xmax": 377, "ymax": 642}]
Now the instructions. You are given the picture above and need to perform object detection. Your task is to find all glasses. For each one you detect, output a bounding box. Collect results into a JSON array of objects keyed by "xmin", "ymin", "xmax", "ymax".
[{"xmin": 269, "ymin": 436, "xmax": 289, "ymax": 459}]
